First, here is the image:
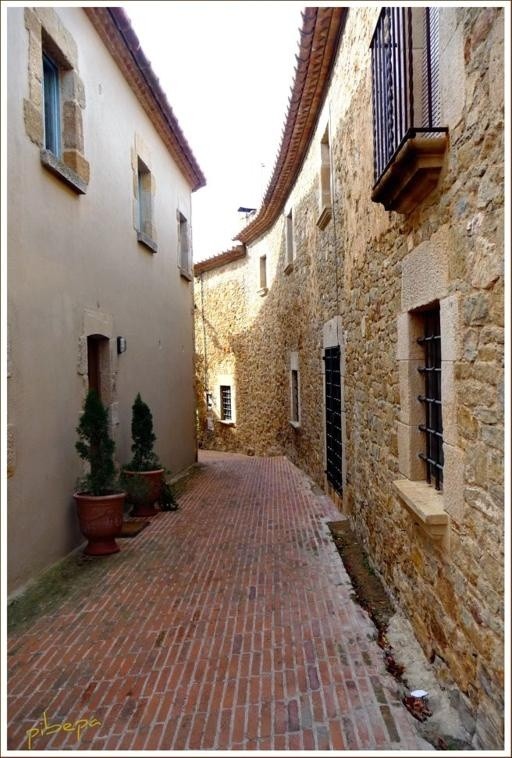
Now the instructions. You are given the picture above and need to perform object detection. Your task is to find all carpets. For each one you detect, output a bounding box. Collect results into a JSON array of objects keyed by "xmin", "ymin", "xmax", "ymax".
[{"xmin": 116, "ymin": 521, "xmax": 149, "ymax": 538}]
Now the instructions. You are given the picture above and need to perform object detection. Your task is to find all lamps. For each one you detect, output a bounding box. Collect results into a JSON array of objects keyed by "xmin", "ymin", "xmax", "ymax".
[{"xmin": 117, "ymin": 336, "xmax": 126, "ymax": 353}]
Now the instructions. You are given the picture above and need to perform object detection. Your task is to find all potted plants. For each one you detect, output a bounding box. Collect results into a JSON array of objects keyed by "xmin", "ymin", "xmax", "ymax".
[
  {"xmin": 74, "ymin": 387, "xmax": 128, "ymax": 556},
  {"xmin": 119, "ymin": 392, "xmax": 179, "ymax": 517}
]
